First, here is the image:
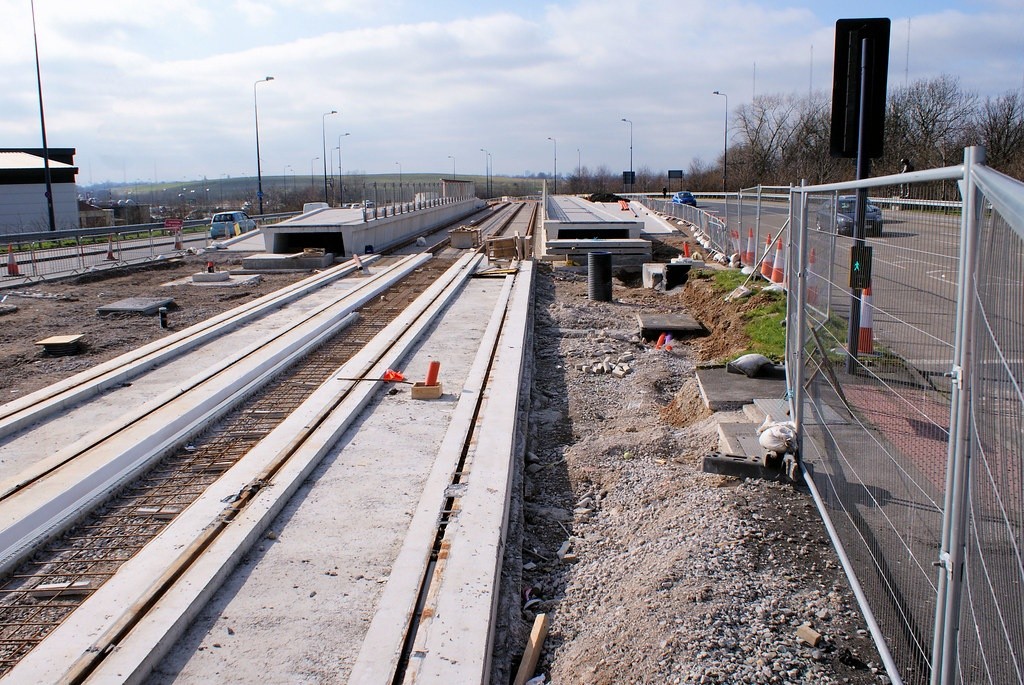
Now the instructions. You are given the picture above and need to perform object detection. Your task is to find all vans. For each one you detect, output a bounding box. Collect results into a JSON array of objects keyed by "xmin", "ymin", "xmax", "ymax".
[{"xmin": 303, "ymin": 203, "xmax": 329, "ymax": 214}]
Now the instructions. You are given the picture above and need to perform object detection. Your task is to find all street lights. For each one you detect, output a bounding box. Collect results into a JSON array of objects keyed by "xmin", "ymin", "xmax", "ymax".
[
  {"xmin": 548, "ymin": 137, "xmax": 556, "ymax": 194},
  {"xmin": 621, "ymin": 119, "xmax": 633, "ymax": 198},
  {"xmin": 713, "ymin": 91, "xmax": 728, "ymax": 193},
  {"xmin": 396, "ymin": 162, "xmax": 401, "ymax": 187},
  {"xmin": 135, "ymin": 171, "xmax": 264, "ymax": 206},
  {"xmin": 578, "ymin": 149, "xmax": 580, "ymax": 182},
  {"xmin": 254, "ymin": 77, "xmax": 274, "ymax": 214},
  {"xmin": 448, "ymin": 156, "xmax": 456, "ymax": 180},
  {"xmin": 481, "ymin": 149, "xmax": 488, "ymax": 198},
  {"xmin": 311, "ymin": 110, "xmax": 351, "ymax": 203},
  {"xmin": 284, "ymin": 165, "xmax": 297, "ymax": 198},
  {"xmin": 488, "ymin": 153, "xmax": 492, "ymax": 198}
]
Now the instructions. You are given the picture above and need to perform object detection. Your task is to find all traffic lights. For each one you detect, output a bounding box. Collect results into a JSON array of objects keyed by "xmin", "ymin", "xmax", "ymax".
[{"xmin": 851, "ymin": 246, "xmax": 872, "ymax": 290}]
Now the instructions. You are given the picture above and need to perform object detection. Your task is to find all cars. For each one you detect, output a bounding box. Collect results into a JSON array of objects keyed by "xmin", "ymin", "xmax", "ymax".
[
  {"xmin": 346, "ymin": 201, "xmax": 375, "ymax": 209},
  {"xmin": 816, "ymin": 195, "xmax": 883, "ymax": 236},
  {"xmin": 211, "ymin": 211, "xmax": 256, "ymax": 238},
  {"xmin": 672, "ymin": 192, "xmax": 697, "ymax": 208}
]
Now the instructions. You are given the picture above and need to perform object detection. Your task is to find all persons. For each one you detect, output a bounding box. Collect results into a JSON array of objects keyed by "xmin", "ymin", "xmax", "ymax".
[
  {"xmin": 900, "ymin": 159, "xmax": 912, "ymax": 198},
  {"xmin": 663, "ymin": 186, "xmax": 667, "ymax": 199}
]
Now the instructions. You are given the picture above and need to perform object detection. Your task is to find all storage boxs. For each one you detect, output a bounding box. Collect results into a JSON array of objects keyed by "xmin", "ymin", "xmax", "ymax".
[{"xmin": 410, "ymin": 381, "xmax": 443, "ymax": 400}]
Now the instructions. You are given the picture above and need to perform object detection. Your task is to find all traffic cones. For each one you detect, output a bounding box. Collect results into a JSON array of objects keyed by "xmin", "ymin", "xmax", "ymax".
[
  {"xmin": 760, "ymin": 233, "xmax": 773, "ymax": 278},
  {"xmin": 770, "ymin": 237, "xmax": 784, "ymax": 282},
  {"xmin": 806, "ymin": 248, "xmax": 819, "ymax": 305},
  {"xmin": 102, "ymin": 236, "xmax": 119, "ymax": 261},
  {"xmin": 4, "ymin": 245, "xmax": 25, "ymax": 277},
  {"xmin": 729, "ymin": 228, "xmax": 745, "ymax": 254},
  {"xmin": 856, "ymin": 288, "xmax": 877, "ymax": 354},
  {"xmin": 745, "ymin": 228, "xmax": 755, "ymax": 265},
  {"xmin": 170, "ymin": 229, "xmax": 185, "ymax": 250}
]
